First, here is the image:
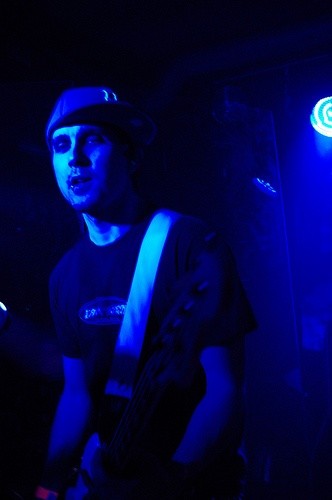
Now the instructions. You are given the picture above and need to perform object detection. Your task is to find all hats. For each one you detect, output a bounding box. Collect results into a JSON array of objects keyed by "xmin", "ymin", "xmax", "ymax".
[{"xmin": 45, "ymin": 87, "xmax": 157, "ymax": 173}]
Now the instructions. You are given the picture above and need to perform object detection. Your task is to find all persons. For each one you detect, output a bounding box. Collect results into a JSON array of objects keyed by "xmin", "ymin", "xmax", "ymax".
[{"xmin": 31, "ymin": 85, "xmax": 257, "ymax": 500}]
